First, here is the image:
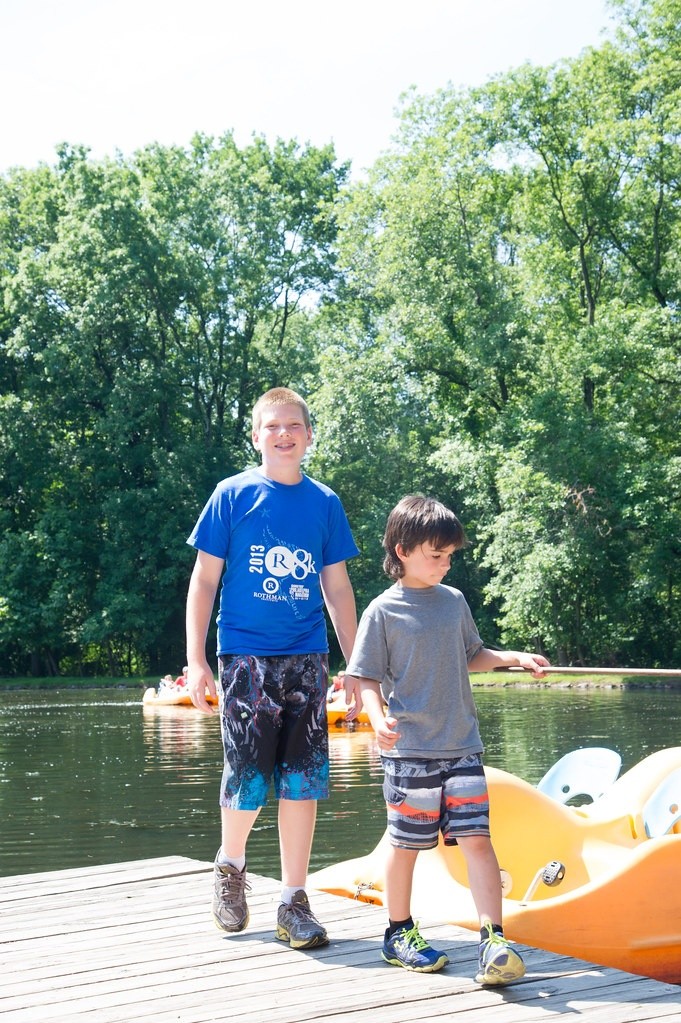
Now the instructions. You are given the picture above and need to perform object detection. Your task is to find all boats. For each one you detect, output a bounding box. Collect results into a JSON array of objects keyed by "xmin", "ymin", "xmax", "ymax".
[
  {"xmin": 306, "ymin": 746, "xmax": 681, "ymax": 986},
  {"xmin": 143, "ymin": 687, "xmax": 219, "ymax": 706},
  {"xmin": 325, "ymin": 689, "xmax": 389, "ymax": 724}
]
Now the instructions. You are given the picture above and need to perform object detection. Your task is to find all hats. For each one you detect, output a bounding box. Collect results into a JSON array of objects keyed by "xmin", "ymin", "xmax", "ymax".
[
  {"xmin": 338, "ymin": 671, "xmax": 346, "ymax": 677},
  {"xmin": 182, "ymin": 666, "xmax": 188, "ymax": 672}
]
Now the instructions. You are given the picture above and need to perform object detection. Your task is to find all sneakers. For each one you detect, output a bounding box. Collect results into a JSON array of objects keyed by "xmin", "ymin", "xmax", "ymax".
[
  {"xmin": 212, "ymin": 845, "xmax": 252, "ymax": 933},
  {"xmin": 380, "ymin": 921, "xmax": 450, "ymax": 973},
  {"xmin": 473, "ymin": 920, "xmax": 526, "ymax": 985},
  {"xmin": 275, "ymin": 890, "xmax": 330, "ymax": 950}
]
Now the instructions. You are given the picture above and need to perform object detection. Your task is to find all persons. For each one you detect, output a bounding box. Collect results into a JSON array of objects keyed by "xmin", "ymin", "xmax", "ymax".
[
  {"xmin": 346, "ymin": 496, "xmax": 552, "ymax": 984},
  {"xmin": 157, "ymin": 666, "xmax": 188, "ymax": 696},
  {"xmin": 185, "ymin": 386, "xmax": 360, "ymax": 950},
  {"xmin": 324, "ymin": 669, "xmax": 346, "ymax": 703}
]
